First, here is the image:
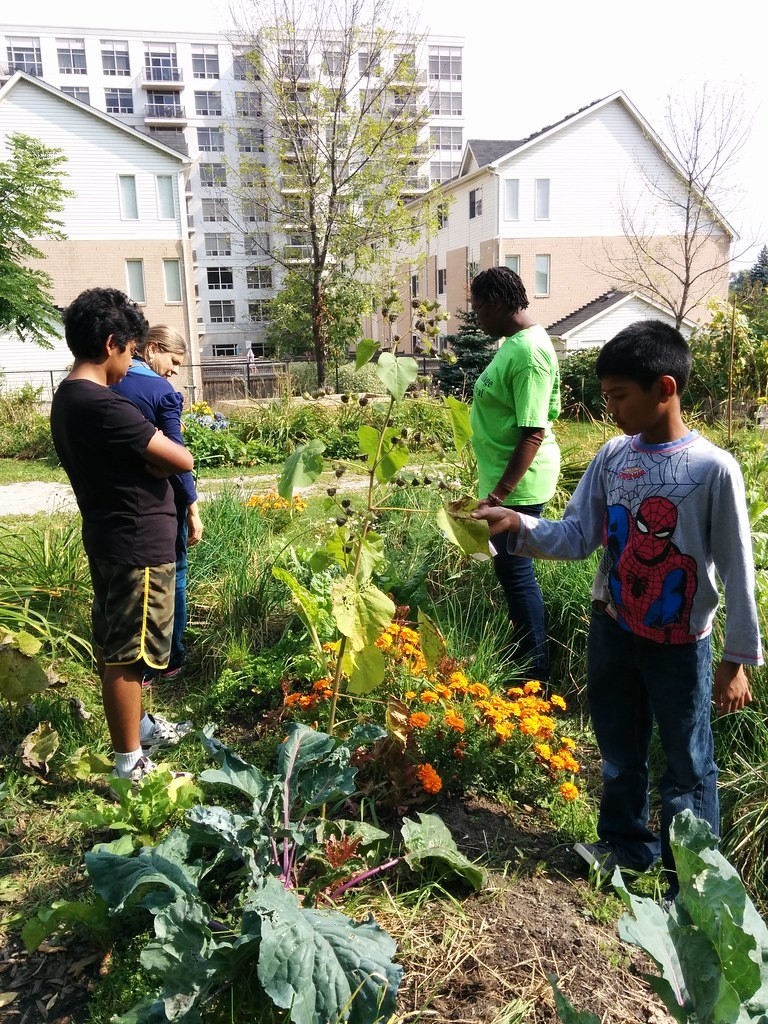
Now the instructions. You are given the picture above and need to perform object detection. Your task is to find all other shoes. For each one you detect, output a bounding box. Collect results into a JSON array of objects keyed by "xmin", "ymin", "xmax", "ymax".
[
  {"xmin": 142, "ymin": 678, "xmax": 154, "ymax": 687},
  {"xmin": 163, "ymin": 668, "xmax": 182, "ymax": 678}
]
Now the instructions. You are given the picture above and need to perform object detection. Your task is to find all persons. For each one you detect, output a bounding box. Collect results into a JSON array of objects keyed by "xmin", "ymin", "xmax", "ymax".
[
  {"xmin": 110, "ymin": 324, "xmax": 203, "ymax": 687},
  {"xmin": 470, "ymin": 320, "xmax": 762, "ymax": 910},
  {"xmin": 51, "ymin": 287, "xmax": 194, "ymax": 804},
  {"xmin": 470, "ymin": 266, "xmax": 561, "ymax": 695}
]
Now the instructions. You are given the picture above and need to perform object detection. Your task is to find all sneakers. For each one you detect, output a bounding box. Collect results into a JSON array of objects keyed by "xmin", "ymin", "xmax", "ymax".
[
  {"xmin": 108, "ymin": 756, "xmax": 195, "ymax": 802},
  {"xmin": 141, "ymin": 711, "xmax": 195, "ymax": 749},
  {"xmin": 573, "ymin": 839, "xmax": 626, "ymax": 878}
]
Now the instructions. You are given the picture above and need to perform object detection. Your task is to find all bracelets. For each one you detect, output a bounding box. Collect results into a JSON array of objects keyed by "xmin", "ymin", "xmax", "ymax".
[{"xmin": 488, "ymin": 493, "xmax": 503, "ymax": 505}]
